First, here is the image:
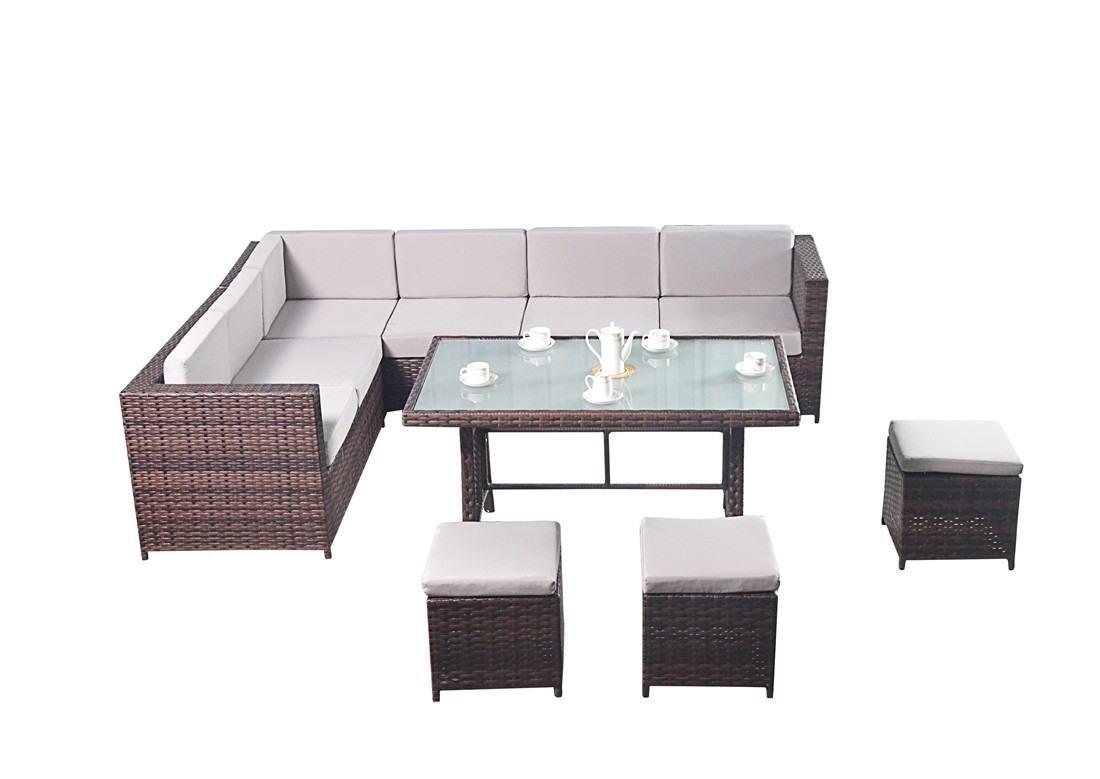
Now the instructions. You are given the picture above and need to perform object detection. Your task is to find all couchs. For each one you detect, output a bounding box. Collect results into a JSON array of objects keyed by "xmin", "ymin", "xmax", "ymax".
[{"xmin": 117, "ymin": 224, "xmax": 829, "ymax": 561}]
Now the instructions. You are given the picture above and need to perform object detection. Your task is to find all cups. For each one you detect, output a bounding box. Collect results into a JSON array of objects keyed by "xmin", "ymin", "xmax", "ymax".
[{"xmin": 458, "ymin": 322, "xmax": 774, "ymax": 405}]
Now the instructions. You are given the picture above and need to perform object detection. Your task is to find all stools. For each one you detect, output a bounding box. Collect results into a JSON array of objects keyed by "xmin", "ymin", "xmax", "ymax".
[
  {"xmin": 421, "ymin": 519, "xmax": 565, "ymax": 703},
  {"xmin": 879, "ymin": 419, "xmax": 1023, "ymax": 571},
  {"xmin": 638, "ymin": 514, "xmax": 781, "ymax": 699}
]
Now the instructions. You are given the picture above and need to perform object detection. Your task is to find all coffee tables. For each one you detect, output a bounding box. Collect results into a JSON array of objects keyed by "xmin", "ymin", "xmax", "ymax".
[{"xmin": 399, "ymin": 333, "xmax": 800, "ymax": 519}]
